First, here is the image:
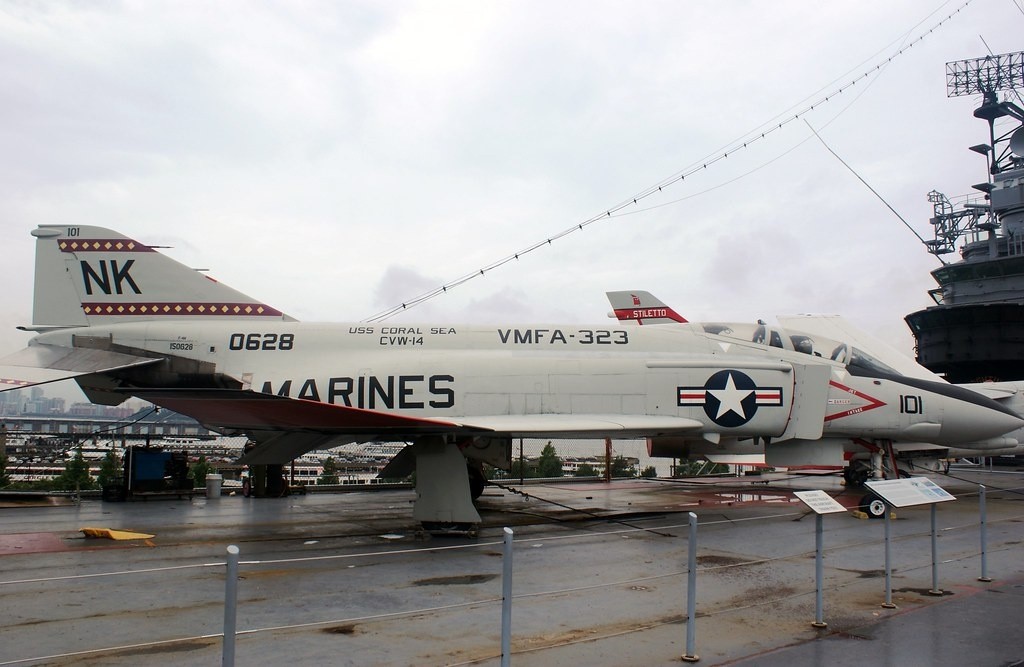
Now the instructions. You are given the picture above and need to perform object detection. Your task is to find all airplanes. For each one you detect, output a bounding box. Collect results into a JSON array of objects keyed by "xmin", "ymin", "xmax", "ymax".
[{"xmin": 0, "ymin": 224, "xmax": 1023, "ymax": 538}]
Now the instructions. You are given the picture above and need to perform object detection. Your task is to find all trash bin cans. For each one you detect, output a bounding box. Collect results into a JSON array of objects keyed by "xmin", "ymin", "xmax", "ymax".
[{"xmin": 206, "ymin": 474, "xmax": 222, "ymax": 499}]
